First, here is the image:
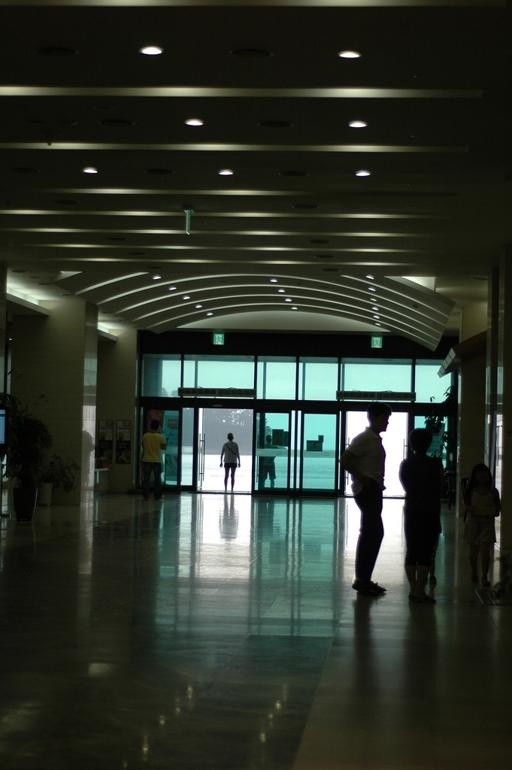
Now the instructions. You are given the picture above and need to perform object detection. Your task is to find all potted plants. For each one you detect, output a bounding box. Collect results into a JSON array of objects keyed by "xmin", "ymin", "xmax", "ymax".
[{"xmin": 0, "ymin": 391, "xmax": 75, "ymax": 521}]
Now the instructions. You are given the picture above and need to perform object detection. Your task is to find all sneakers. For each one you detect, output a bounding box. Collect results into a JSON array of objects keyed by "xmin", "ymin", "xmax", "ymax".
[
  {"xmin": 352, "ymin": 578, "xmax": 387, "ymax": 596},
  {"xmin": 407, "ymin": 570, "xmax": 438, "ymax": 605},
  {"xmin": 468, "ymin": 575, "xmax": 493, "ymax": 588}
]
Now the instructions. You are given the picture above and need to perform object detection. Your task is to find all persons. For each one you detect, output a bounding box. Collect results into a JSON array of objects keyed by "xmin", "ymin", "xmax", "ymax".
[
  {"xmin": 220, "ymin": 433, "xmax": 240, "ymax": 493},
  {"xmin": 400, "ymin": 428, "xmax": 442, "ymax": 603},
  {"xmin": 464, "ymin": 463, "xmax": 500, "ymax": 587},
  {"xmin": 341, "ymin": 403, "xmax": 391, "ymax": 595},
  {"xmin": 263, "ymin": 435, "xmax": 278, "ymax": 489},
  {"xmin": 142, "ymin": 420, "xmax": 167, "ymax": 501}
]
